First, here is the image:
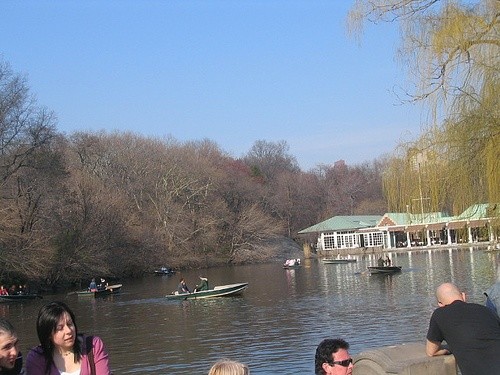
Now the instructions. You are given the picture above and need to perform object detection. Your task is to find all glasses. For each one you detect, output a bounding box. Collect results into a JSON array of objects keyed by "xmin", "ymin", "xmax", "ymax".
[{"xmin": 333, "ymin": 358, "xmax": 352, "ymax": 367}]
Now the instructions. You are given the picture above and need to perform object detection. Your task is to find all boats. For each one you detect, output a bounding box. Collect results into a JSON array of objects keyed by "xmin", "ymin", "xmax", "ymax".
[
  {"xmin": 164, "ymin": 281, "xmax": 249, "ymax": 300},
  {"xmin": 75, "ymin": 284, "xmax": 123, "ymax": 298},
  {"xmin": 322, "ymin": 253, "xmax": 357, "ymax": 265},
  {"xmin": 0, "ymin": 293, "xmax": 44, "ymax": 303},
  {"xmin": 280, "ymin": 258, "xmax": 304, "ymax": 271},
  {"xmin": 366, "ymin": 265, "xmax": 403, "ymax": 275}
]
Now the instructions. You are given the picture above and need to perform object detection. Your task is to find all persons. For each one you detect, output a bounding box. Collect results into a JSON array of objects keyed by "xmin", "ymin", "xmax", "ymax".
[
  {"xmin": 25, "ymin": 301, "xmax": 111, "ymax": 375},
  {"xmin": 193, "ymin": 274, "xmax": 208, "ymax": 291},
  {"xmin": 90, "ymin": 278, "xmax": 98, "ymax": 292},
  {"xmin": 484, "ymin": 282, "xmax": 500, "ymax": 317},
  {"xmin": 385, "ymin": 256, "xmax": 391, "ymax": 266},
  {"xmin": 12, "ymin": 284, "xmax": 29, "ymax": 295},
  {"xmin": 315, "ymin": 339, "xmax": 353, "ymax": 375},
  {"xmin": 208, "ymin": 359, "xmax": 250, "ymax": 375},
  {"xmin": 427, "ymin": 283, "xmax": 500, "ymax": 375},
  {"xmin": 0, "ymin": 286, "xmax": 10, "ymax": 295},
  {"xmin": 286, "ymin": 258, "xmax": 299, "ymax": 266},
  {"xmin": 378, "ymin": 256, "xmax": 385, "ymax": 266},
  {"xmin": 178, "ymin": 278, "xmax": 191, "ymax": 293},
  {"xmin": 99, "ymin": 278, "xmax": 105, "ymax": 286},
  {"xmin": 0, "ymin": 319, "xmax": 23, "ymax": 375}
]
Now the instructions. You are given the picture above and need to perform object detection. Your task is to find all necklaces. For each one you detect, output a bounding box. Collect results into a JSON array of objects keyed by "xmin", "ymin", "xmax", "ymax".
[{"xmin": 65, "ymin": 350, "xmax": 72, "ymax": 356}]
